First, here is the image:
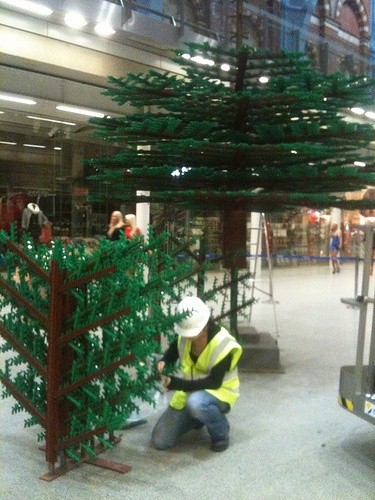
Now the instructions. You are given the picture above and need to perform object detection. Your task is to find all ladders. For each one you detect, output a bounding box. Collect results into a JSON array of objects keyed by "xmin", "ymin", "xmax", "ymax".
[{"xmin": 221, "ymin": 212, "xmax": 281, "ymax": 337}]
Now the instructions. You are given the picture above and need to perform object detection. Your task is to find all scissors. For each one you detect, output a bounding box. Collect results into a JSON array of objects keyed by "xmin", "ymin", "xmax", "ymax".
[{"xmin": 153, "ymin": 369, "xmax": 168, "ymax": 401}]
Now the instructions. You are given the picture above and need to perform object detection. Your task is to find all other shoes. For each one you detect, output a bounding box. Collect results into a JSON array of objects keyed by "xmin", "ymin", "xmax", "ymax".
[{"xmin": 211, "ymin": 437, "xmax": 229, "ymax": 452}]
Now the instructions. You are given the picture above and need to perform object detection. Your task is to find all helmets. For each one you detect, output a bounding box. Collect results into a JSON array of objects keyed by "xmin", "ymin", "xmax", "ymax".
[{"xmin": 173, "ymin": 297, "xmax": 211, "ymax": 337}]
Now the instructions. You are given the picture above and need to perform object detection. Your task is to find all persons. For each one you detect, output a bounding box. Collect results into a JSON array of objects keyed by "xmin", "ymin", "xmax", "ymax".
[
  {"xmin": 329, "ymin": 224, "xmax": 342, "ymax": 274},
  {"xmin": 21, "ymin": 203, "xmax": 48, "ymax": 246},
  {"xmin": 105, "ymin": 211, "xmax": 142, "ymax": 277},
  {"xmin": 150, "ymin": 296, "xmax": 244, "ymax": 451}
]
